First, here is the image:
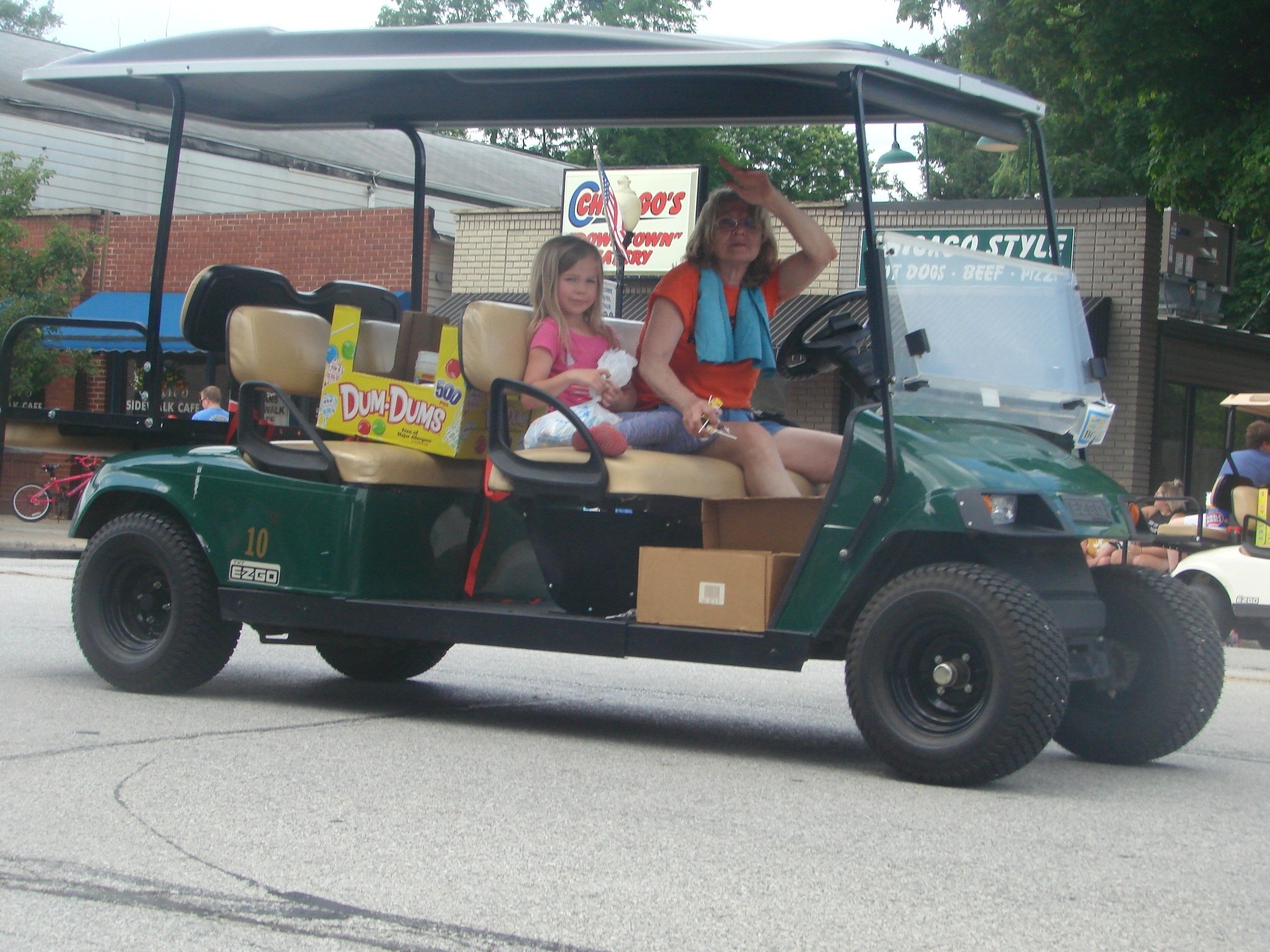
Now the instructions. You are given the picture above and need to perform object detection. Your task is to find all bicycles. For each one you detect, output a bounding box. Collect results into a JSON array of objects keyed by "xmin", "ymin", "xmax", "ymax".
[{"xmin": 10, "ymin": 449, "xmax": 99, "ymax": 522}]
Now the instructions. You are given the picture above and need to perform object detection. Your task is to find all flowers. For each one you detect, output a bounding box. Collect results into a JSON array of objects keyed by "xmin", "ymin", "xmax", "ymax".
[{"xmin": 133, "ymin": 363, "xmax": 187, "ymax": 393}]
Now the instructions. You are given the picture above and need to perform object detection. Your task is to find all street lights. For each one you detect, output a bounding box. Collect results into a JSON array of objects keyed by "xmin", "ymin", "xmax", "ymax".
[{"xmin": 611, "ymin": 173, "xmax": 640, "ymax": 319}]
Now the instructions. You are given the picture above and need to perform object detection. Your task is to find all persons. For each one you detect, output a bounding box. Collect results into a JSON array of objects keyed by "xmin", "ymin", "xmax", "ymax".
[
  {"xmin": 520, "ymin": 235, "xmax": 721, "ymax": 454},
  {"xmin": 632, "ymin": 154, "xmax": 846, "ymax": 499},
  {"xmin": 1211, "ymin": 420, "xmax": 1270, "ymax": 527},
  {"xmin": 191, "ymin": 385, "xmax": 229, "ymax": 424},
  {"xmin": 1080, "ymin": 478, "xmax": 1188, "ymax": 572}
]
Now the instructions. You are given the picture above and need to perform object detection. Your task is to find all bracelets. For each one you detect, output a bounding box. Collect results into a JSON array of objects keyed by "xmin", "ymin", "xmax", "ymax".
[{"xmin": 1141, "ymin": 546, "xmax": 1142, "ymax": 554}]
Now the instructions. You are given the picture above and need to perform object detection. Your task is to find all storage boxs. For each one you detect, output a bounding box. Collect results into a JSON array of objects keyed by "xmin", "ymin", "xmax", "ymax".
[
  {"xmin": 310, "ymin": 306, "xmax": 530, "ymax": 457},
  {"xmin": 636, "ymin": 495, "xmax": 824, "ymax": 634}
]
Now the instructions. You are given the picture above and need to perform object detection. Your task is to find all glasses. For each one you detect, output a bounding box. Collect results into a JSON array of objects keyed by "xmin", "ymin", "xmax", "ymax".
[
  {"xmin": 715, "ymin": 216, "xmax": 762, "ymax": 231},
  {"xmin": 1154, "ymin": 492, "xmax": 1161, "ymax": 500},
  {"xmin": 199, "ymin": 398, "xmax": 205, "ymax": 402}
]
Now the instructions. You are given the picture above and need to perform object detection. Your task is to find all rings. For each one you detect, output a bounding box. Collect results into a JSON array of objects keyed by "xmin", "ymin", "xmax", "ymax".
[{"xmin": 1129, "ymin": 550, "xmax": 1130, "ymax": 552}]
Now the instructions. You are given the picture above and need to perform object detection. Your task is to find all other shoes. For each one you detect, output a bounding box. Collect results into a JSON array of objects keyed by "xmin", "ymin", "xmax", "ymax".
[{"xmin": 571, "ymin": 424, "xmax": 628, "ymax": 456}]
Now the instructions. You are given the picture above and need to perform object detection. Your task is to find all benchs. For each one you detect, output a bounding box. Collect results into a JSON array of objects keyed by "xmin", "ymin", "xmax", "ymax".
[
  {"xmin": 1153, "ymin": 473, "xmax": 1270, "ymax": 552},
  {"xmin": 225, "ymin": 307, "xmax": 485, "ymax": 488},
  {"xmin": 1228, "ymin": 486, "xmax": 1270, "ymax": 560},
  {"xmin": 464, "ymin": 298, "xmax": 826, "ymax": 496}
]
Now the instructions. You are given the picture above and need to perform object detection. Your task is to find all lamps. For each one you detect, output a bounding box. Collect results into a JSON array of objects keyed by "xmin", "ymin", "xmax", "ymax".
[
  {"xmin": 878, "ymin": 120, "xmax": 931, "ymax": 200},
  {"xmin": 977, "ymin": 125, "xmax": 1035, "ymax": 199}
]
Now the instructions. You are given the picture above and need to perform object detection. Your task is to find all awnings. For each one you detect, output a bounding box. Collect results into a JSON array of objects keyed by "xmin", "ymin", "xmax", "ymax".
[{"xmin": 39, "ymin": 291, "xmax": 212, "ymax": 355}]
